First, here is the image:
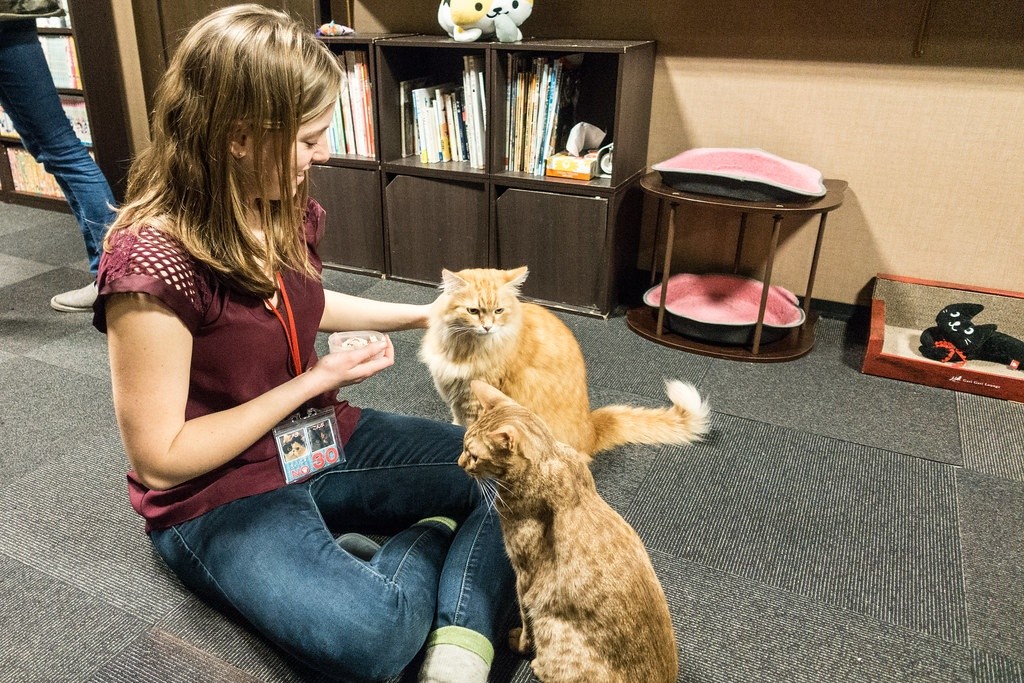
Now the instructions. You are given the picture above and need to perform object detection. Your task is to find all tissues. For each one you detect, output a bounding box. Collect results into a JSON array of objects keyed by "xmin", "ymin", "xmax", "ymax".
[{"xmin": 545, "ymin": 122, "xmax": 607, "ymax": 181}]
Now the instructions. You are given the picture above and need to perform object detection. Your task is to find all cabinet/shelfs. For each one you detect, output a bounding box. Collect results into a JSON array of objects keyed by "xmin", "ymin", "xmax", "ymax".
[
  {"xmin": 0, "ymin": 0, "xmax": 132, "ymax": 215},
  {"xmin": 305, "ymin": 33, "xmax": 659, "ymax": 319}
]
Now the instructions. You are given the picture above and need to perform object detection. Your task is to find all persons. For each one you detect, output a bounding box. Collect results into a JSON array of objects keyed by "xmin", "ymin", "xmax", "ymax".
[
  {"xmin": 93, "ymin": 4, "xmax": 520, "ymax": 682},
  {"xmin": 0, "ymin": 1, "xmax": 119, "ymax": 311}
]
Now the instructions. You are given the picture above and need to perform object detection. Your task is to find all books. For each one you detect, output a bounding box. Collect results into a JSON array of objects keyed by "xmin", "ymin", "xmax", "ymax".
[
  {"xmin": 0, "ymin": 0, "xmax": 96, "ymax": 199},
  {"xmin": 504, "ymin": 52, "xmax": 584, "ymax": 176},
  {"xmin": 399, "ymin": 55, "xmax": 487, "ymax": 170},
  {"xmin": 327, "ymin": 49, "xmax": 376, "ymax": 158}
]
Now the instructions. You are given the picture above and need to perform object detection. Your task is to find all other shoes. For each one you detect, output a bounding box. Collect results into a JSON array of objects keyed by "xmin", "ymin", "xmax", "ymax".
[{"xmin": 50, "ymin": 280, "xmax": 99, "ymax": 313}]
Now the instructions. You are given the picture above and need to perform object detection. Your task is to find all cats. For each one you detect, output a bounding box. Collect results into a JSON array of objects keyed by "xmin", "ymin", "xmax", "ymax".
[{"xmin": 418, "ymin": 265, "xmax": 716, "ymax": 682}]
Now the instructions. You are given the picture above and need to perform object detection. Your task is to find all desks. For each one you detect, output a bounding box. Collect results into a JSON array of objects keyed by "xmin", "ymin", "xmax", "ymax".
[{"xmin": 625, "ymin": 172, "xmax": 848, "ymax": 364}]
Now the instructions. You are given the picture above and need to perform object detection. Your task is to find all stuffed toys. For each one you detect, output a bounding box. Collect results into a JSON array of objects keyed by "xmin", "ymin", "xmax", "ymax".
[
  {"xmin": 919, "ymin": 302, "xmax": 1024, "ymax": 370},
  {"xmin": 437, "ymin": 1, "xmax": 534, "ymax": 43}
]
